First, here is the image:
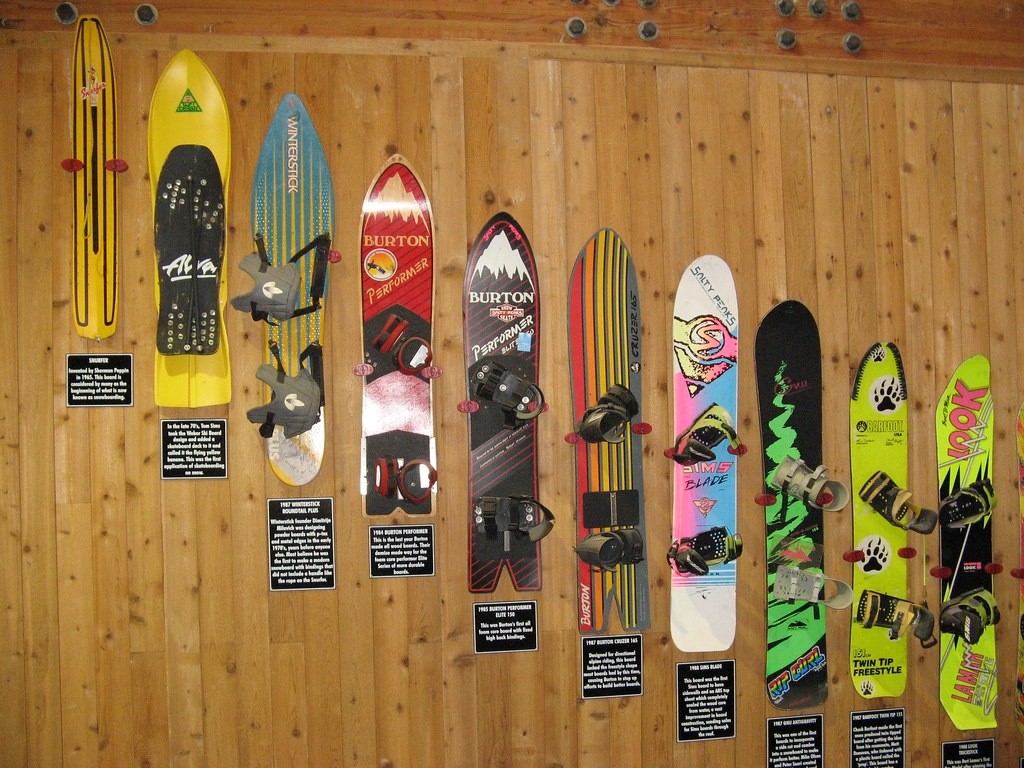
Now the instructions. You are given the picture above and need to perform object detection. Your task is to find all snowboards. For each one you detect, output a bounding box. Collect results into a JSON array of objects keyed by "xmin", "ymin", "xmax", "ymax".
[{"xmin": 71, "ymin": 11, "xmax": 1023, "ymax": 732}]
